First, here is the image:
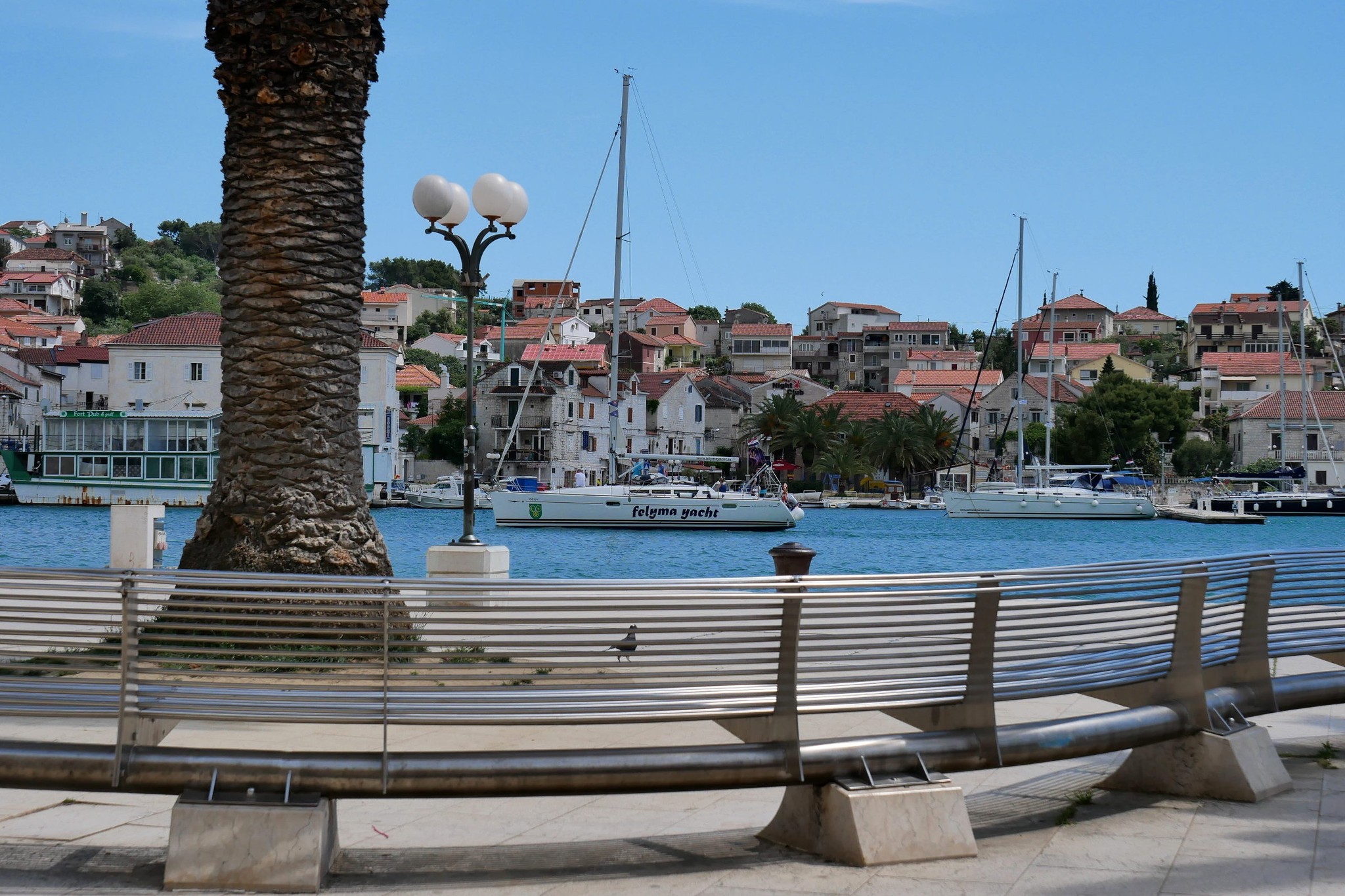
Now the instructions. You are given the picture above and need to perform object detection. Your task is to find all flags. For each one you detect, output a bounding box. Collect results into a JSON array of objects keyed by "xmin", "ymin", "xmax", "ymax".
[
  {"xmin": 748, "ymin": 436, "xmax": 759, "ymax": 447},
  {"xmin": 1126, "ymin": 459, "xmax": 1134, "ymax": 465},
  {"xmin": 1110, "ymin": 455, "xmax": 1120, "ymax": 461}
]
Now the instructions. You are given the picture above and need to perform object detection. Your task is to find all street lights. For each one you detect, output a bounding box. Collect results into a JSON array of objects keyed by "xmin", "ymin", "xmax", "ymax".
[
  {"xmin": 485, "ymin": 453, "xmax": 500, "ymax": 475},
  {"xmin": 412, "ymin": 172, "xmax": 530, "ymax": 575}
]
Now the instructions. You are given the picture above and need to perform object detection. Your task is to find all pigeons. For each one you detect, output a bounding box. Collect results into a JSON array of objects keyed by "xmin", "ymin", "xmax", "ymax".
[{"xmin": 603, "ymin": 625, "xmax": 638, "ymax": 662}]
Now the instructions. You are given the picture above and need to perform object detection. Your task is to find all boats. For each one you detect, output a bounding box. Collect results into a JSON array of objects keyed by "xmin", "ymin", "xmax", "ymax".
[
  {"xmin": 879, "ymin": 480, "xmax": 912, "ymax": 510},
  {"xmin": 828, "ymin": 502, "xmax": 850, "ymax": 509},
  {"xmin": 366, "ymin": 475, "xmax": 494, "ymax": 510},
  {"xmin": 916, "ymin": 490, "xmax": 948, "ymax": 510}
]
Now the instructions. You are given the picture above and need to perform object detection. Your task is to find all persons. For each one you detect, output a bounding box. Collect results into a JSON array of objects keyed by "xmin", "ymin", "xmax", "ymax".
[
  {"xmin": 658, "ymin": 459, "xmax": 666, "ymax": 475},
  {"xmin": 719, "ymin": 479, "xmax": 726, "ymax": 493},
  {"xmin": 573, "ymin": 469, "xmax": 586, "ymax": 488},
  {"xmin": 751, "ymin": 471, "xmax": 787, "ymax": 501}
]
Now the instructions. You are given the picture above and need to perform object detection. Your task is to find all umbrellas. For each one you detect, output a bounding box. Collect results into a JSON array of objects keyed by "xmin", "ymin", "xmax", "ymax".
[{"xmin": 769, "ymin": 459, "xmax": 802, "ymax": 471}]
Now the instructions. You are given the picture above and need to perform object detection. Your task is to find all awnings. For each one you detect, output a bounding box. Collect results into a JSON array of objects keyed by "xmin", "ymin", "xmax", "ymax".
[{"xmin": 683, "ymin": 464, "xmax": 722, "ymax": 471}]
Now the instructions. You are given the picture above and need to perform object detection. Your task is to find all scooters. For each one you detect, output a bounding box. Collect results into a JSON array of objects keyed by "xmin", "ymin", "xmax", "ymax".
[{"xmin": 920, "ymin": 483, "xmax": 933, "ymax": 500}]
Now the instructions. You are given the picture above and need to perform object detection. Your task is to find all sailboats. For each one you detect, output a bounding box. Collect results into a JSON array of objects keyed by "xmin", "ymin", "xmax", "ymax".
[
  {"xmin": 936, "ymin": 214, "xmax": 1161, "ymax": 519},
  {"xmin": 1187, "ymin": 292, "xmax": 1345, "ymax": 516},
  {"xmin": 479, "ymin": 71, "xmax": 806, "ymax": 531},
  {"xmin": 702, "ymin": 428, "xmax": 720, "ymax": 468}
]
